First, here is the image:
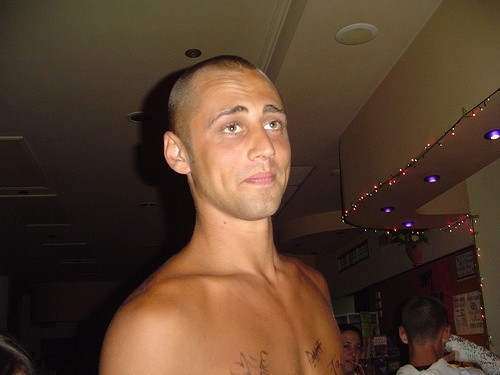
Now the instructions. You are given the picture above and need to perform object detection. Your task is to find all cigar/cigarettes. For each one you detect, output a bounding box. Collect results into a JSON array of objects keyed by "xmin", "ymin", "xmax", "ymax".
[{"xmin": 350, "ymin": 360, "xmax": 362, "ymax": 368}]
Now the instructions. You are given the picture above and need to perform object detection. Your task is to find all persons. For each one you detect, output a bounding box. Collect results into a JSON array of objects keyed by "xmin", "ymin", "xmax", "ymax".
[
  {"xmin": 99, "ymin": 55, "xmax": 345, "ymax": 375},
  {"xmin": 338, "ymin": 323, "xmax": 362, "ymax": 375},
  {"xmin": 387, "ymin": 295, "xmax": 485, "ymax": 375}
]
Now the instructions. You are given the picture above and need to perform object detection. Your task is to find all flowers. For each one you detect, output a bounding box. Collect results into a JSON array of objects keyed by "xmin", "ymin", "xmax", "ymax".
[{"xmin": 390, "ymin": 231, "xmax": 430, "ymax": 248}]
